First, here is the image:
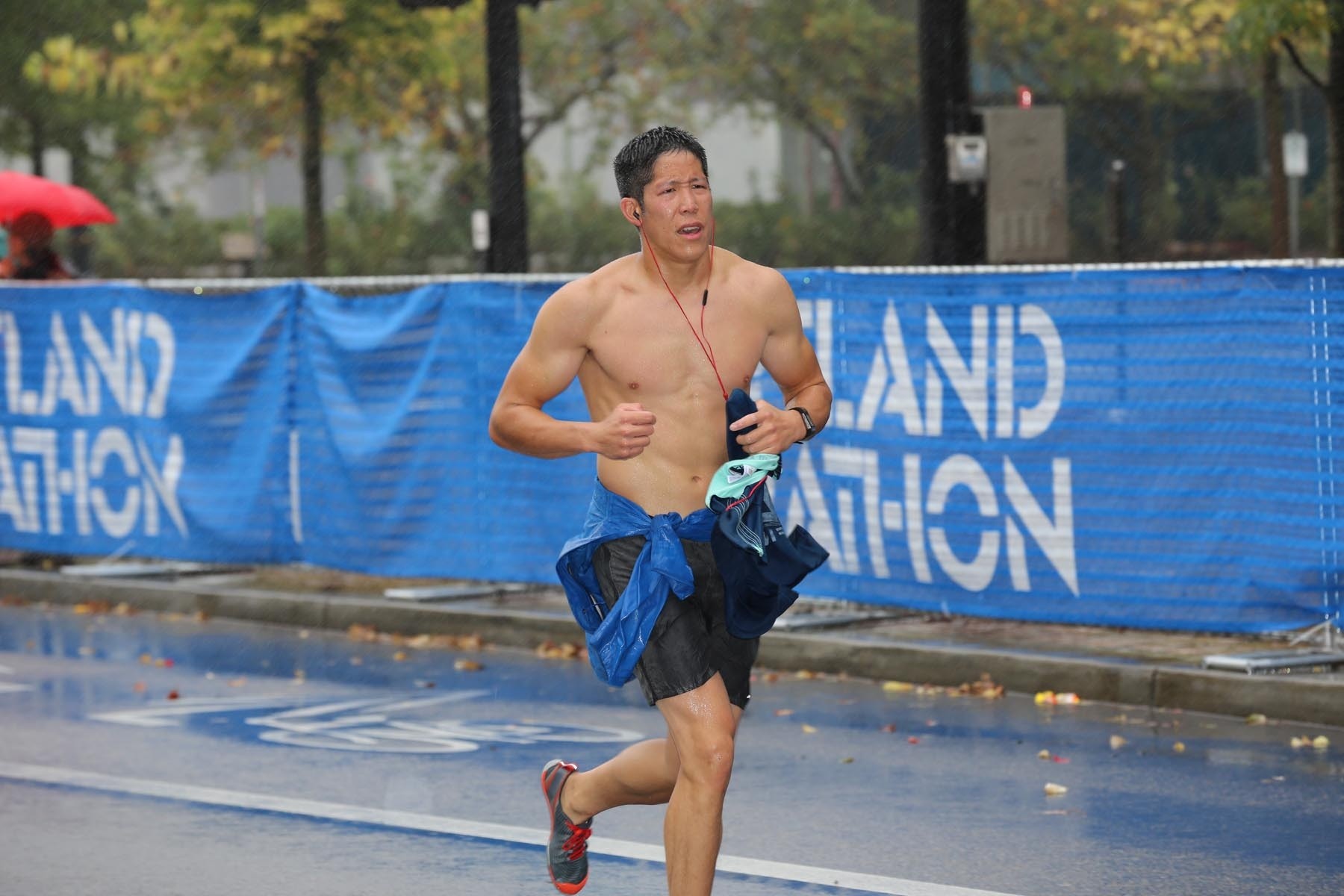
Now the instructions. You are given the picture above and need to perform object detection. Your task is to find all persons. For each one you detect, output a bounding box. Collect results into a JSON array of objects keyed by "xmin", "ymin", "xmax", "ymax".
[
  {"xmin": 0, "ymin": 209, "xmax": 82, "ymax": 281},
  {"xmin": 486, "ymin": 126, "xmax": 833, "ymax": 896}
]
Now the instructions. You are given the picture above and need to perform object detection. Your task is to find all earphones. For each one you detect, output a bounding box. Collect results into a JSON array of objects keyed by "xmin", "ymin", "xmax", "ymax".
[{"xmin": 632, "ymin": 210, "xmax": 640, "ymax": 219}]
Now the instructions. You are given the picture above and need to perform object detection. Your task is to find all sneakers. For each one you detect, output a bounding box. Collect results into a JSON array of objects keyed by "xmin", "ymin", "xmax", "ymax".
[{"xmin": 541, "ymin": 758, "xmax": 592, "ymax": 895}]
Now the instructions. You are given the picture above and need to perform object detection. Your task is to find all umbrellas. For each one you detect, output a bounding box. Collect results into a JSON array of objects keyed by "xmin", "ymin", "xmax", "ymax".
[{"xmin": 0, "ymin": 168, "xmax": 116, "ymax": 231}]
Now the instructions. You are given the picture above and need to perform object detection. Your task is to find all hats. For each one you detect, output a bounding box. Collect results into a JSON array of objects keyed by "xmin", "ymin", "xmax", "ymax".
[{"xmin": 3, "ymin": 211, "xmax": 52, "ymax": 241}]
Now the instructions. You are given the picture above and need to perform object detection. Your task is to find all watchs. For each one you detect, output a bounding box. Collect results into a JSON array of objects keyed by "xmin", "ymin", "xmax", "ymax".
[{"xmin": 787, "ymin": 406, "xmax": 817, "ymax": 445}]
